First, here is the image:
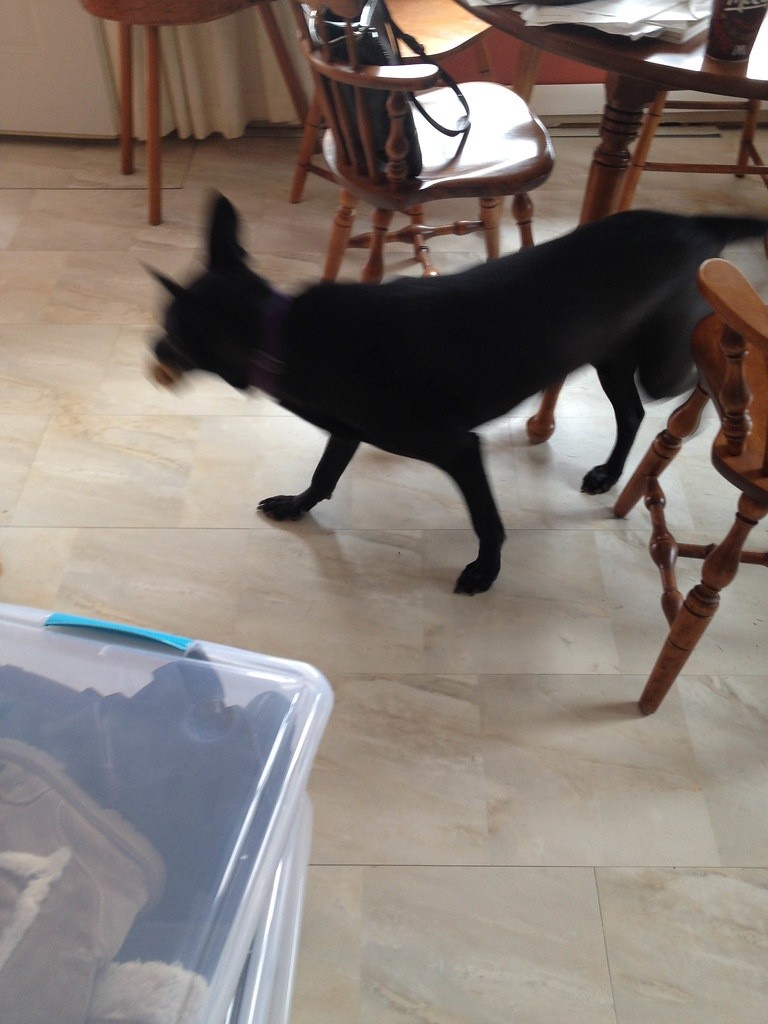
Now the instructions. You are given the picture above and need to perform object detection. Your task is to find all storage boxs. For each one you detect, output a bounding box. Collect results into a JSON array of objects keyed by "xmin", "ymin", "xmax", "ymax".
[{"xmin": 1, "ymin": 598, "xmax": 334, "ymax": 1024}]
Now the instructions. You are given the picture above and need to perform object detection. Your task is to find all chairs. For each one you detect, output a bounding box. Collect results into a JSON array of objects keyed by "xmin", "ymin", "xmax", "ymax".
[
  {"xmin": 78, "ymin": 0, "xmax": 557, "ymax": 289},
  {"xmin": 610, "ymin": 259, "xmax": 768, "ymax": 716}
]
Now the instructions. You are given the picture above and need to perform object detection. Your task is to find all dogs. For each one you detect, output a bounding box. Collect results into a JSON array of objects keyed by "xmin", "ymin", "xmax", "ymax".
[{"xmin": 136, "ymin": 188, "xmax": 721, "ymax": 597}]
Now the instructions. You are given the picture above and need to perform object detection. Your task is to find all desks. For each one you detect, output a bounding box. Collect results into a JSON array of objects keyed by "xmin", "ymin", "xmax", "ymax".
[{"xmin": 457, "ymin": 0, "xmax": 768, "ymax": 226}]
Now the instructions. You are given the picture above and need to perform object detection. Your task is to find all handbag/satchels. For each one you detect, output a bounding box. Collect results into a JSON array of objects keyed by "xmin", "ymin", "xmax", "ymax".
[{"xmin": 308, "ymin": 0, "xmax": 469, "ymax": 179}]
